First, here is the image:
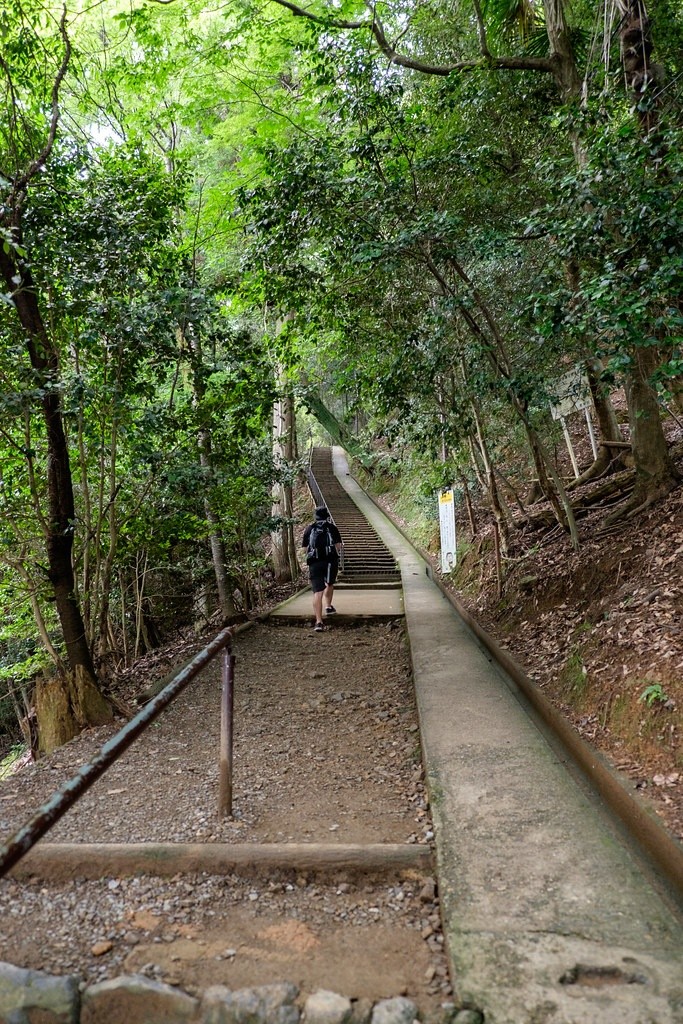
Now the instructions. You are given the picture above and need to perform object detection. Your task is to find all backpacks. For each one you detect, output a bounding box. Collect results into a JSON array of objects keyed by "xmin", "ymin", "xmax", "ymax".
[{"xmin": 307, "ymin": 522, "xmax": 333, "ymax": 565}]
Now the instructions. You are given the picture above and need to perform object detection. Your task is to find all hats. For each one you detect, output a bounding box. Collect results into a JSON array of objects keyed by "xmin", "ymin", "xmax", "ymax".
[{"xmin": 313, "ymin": 506, "xmax": 329, "ymax": 519}]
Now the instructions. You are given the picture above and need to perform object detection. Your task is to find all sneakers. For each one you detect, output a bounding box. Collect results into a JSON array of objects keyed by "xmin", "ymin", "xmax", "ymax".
[
  {"xmin": 314, "ymin": 622, "xmax": 326, "ymax": 632},
  {"xmin": 325, "ymin": 605, "xmax": 336, "ymax": 615}
]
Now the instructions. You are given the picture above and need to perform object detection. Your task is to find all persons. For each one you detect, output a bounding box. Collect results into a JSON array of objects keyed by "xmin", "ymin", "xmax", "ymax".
[{"xmin": 301, "ymin": 506, "xmax": 342, "ymax": 631}]
